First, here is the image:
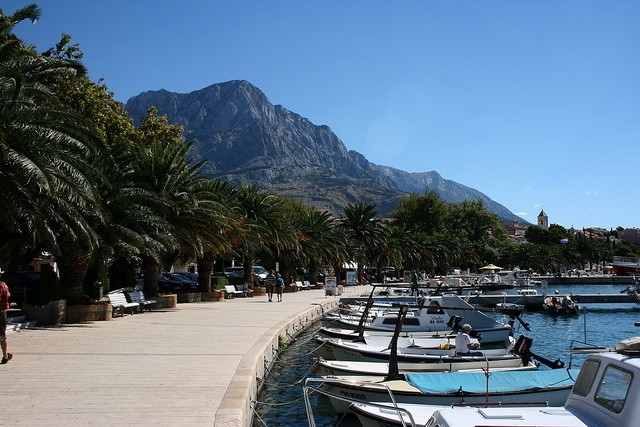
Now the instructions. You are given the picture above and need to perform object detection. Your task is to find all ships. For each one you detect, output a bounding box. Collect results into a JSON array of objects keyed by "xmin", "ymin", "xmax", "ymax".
[{"xmin": 609, "ymin": 255, "xmax": 640, "ymax": 275}]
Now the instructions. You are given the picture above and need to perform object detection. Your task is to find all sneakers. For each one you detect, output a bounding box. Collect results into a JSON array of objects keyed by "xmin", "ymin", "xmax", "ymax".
[{"xmin": 2, "ymin": 353, "xmax": 12, "ymax": 364}]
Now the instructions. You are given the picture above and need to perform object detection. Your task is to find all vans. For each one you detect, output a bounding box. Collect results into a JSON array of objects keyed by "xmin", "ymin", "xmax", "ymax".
[{"xmin": 224, "ymin": 265, "xmax": 269, "ymax": 287}]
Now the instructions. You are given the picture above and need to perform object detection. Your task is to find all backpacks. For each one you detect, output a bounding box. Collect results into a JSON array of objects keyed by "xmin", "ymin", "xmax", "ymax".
[{"xmin": 277, "ymin": 278, "xmax": 282, "ymax": 286}]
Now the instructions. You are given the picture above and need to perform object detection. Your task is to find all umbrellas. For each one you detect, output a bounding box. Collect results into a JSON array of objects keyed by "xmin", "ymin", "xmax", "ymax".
[{"xmin": 478, "ymin": 264, "xmax": 505, "ymax": 271}]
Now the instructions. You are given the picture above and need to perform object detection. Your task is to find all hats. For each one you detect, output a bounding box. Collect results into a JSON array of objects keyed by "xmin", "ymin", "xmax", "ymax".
[
  {"xmin": 0, "ymin": 268, "xmax": 5, "ymax": 274},
  {"xmin": 277, "ymin": 273, "xmax": 281, "ymax": 277},
  {"xmin": 269, "ymin": 269, "xmax": 272, "ymax": 273}
]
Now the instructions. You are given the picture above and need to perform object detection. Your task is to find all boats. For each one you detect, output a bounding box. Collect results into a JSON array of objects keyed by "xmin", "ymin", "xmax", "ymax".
[
  {"xmin": 542, "ymin": 297, "xmax": 580, "ymax": 316},
  {"xmin": 303, "ymin": 336, "xmax": 640, "ymax": 427},
  {"xmin": 320, "ymin": 303, "xmax": 599, "ymax": 418},
  {"xmin": 312, "ymin": 335, "xmax": 566, "ymax": 378},
  {"xmin": 308, "ymin": 270, "xmax": 542, "ymax": 360}
]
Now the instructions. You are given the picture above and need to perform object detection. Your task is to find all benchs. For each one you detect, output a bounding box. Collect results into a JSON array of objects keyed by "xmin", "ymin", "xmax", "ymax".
[
  {"xmin": 223, "ymin": 285, "xmax": 244, "ymax": 300},
  {"xmin": 235, "ymin": 282, "xmax": 255, "ymax": 298},
  {"xmin": 127, "ymin": 290, "xmax": 157, "ymax": 313},
  {"xmin": 294, "ymin": 281, "xmax": 309, "ymax": 291},
  {"xmin": 303, "ymin": 280, "xmax": 316, "ymax": 289},
  {"xmin": 106, "ymin": 292, "xmax": 140, "ymax": 319}
]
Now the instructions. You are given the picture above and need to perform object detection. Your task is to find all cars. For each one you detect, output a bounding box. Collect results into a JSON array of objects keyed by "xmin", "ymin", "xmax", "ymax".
[
  {"xmin": 135, "ymin": 271, "xmax": 198, "ymax": 293},
  {"xmin": 214, "ymin": 272, "xmax": 237, "ymax": 276}
]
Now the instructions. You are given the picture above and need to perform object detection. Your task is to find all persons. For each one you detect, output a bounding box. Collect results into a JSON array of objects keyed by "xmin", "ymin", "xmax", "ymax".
[
  {"xmin": 243, "ymin": 278, "xmax": 255, "ymax": 298},
  {"xmin": 455, "ymin": 323, "xmax": 483, "ymax": 357},
  {"xmin": 409, "ymin": 269, "xmax": 420, "ymax": 296},
  {"xmin": 266, "ymin": 269, "xmax": 276, "ymax": 302},
  {"xmin": 0, "ymin": 268, "xmax": 14, "ymax": 366},
  {"xmin": 276, "ymin": 273, "xmax": 285, "ymax": 302},
  {"xmin": 361, "ymin": 270, "xmax": 373, "ymax": 287},
  {"xmin": 420, "ymin": 270, "xmax": 430, "ymax": 282}
]
